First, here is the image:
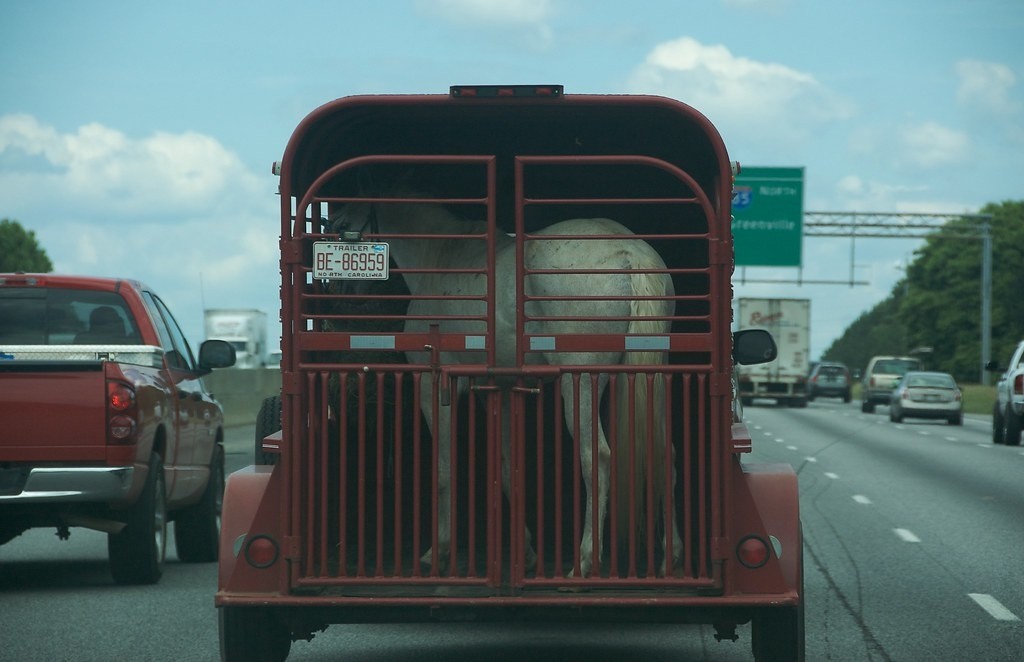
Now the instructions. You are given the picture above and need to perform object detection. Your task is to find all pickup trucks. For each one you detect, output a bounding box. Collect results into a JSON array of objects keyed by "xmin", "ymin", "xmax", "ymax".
[{"xmin": 1, "ymin": 272, "xmax": 237, "ymax": 587}]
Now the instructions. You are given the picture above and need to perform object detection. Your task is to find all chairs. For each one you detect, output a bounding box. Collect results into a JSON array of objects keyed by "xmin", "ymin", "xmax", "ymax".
[{"xmin": 74, "ymin": 306, "xmax": 126, "ymax": 345}]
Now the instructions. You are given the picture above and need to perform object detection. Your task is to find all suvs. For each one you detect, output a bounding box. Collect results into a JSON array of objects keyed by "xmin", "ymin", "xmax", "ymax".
[
  {"xmin": 854, "ymin": 355, "xmax": 925, "ymax": 415},
  {"xmin": 984, "ymin": 339, "xmax": 1024, "ymax": 446},
  {"xmin": 806, "ymin": 362, "xmax": 851, "ymax": 404}
]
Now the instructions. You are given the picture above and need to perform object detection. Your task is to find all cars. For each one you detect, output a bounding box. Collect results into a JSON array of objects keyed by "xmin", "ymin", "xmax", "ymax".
[
  {"xmin": 890, "ymin": 370, "xmax": 965, "ymax": 427},
  {"xmin": 261, "ymin": 349, "xmax": 282, "ymax": 371}
]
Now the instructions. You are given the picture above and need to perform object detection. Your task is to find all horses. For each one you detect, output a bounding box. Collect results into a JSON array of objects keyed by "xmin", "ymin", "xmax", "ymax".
[{"xmin": 313, "ymin": 180, "xmax": 683, "ymax": 594}]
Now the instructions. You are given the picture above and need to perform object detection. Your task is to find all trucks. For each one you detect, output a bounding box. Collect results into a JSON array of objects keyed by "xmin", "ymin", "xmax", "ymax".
[
  {"xmin": 728, "ymin": 296, "xmax": 810, "ymax": 408},
  {"xmin": 202, "ymin": 305, "xmax": 270, "ymax": 371},
  {"xmin": 211, "ymin": 84, "xmax": 809, "ymax": 662}
]
